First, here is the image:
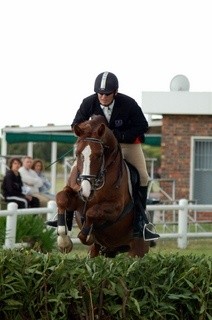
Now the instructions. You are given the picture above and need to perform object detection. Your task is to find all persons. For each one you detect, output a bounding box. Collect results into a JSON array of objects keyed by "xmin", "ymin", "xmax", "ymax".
[
  {"xmin": 46, "ymin": 72, "xmax": 160, "ymax": 239},
  {"xmin": 0, "ymin": 156, "xmax": 56, "ymax": 223}
]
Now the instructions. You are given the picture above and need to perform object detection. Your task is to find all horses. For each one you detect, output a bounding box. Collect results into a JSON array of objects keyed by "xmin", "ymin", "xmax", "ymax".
[{"xmin": 55, "ymin": 114, "xmax": 151, "ymax": 259}]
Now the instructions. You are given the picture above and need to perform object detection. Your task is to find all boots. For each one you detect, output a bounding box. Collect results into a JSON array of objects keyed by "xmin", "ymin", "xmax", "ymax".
[
  {"xmin": 132, "ymin": 185, "xmax": 160, "ymax": 240},
  {"xmin": 46, "ymin": 186, "xmax": 76, "ymax": 230}
]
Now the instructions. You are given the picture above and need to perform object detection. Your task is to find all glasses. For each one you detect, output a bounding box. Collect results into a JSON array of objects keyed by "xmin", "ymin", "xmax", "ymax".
[{"xmin": 99, "ymin": 92, "xmax": 113, "ymax": 96}]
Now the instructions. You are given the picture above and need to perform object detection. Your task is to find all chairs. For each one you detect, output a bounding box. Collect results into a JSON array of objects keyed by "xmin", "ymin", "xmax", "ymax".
[{"xmin": 0, "ymin": 180, "xmax": 28, "ymax": 209}]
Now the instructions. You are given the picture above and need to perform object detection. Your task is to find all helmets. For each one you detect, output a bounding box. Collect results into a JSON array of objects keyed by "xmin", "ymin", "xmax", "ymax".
[{"xmin": 93, "ymin": 71, "xmax": 119, "ymax": 94}]
